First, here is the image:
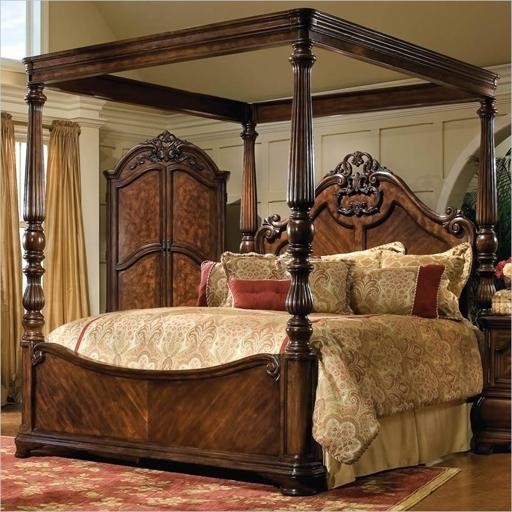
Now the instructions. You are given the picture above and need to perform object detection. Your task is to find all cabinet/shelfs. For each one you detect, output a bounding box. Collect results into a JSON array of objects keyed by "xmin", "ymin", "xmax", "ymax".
[{"xmin": 102, "ymin": 130, "xmax": 231, "ymax": 310}]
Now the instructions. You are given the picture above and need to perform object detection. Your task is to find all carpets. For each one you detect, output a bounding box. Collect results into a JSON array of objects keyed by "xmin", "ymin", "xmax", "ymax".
[{"xmin": 1, "ymin": 437, "xmax": 464, "ymax": 510}]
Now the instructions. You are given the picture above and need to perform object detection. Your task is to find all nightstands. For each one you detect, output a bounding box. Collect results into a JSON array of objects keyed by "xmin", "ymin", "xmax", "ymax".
[{"xmin": 471, "ymin": 314, "xmax": 511, "ymax": 454}]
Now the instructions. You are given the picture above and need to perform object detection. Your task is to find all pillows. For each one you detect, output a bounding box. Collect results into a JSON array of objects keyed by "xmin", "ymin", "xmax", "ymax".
[{"xmin": 197, "ymin": 238, "xmax": 473, "ymax": 325}]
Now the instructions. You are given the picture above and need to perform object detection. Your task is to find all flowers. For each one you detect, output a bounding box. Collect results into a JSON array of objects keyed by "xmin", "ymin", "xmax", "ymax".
[{"xmin": 495, "ymin": 256, "xmax": 512, "ymax": 286}]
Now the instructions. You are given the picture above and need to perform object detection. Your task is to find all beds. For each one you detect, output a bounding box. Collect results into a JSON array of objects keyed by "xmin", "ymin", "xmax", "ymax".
[{"xmin": 13, "ymin": 149, "xmax": 479, "ymax": 496}]
